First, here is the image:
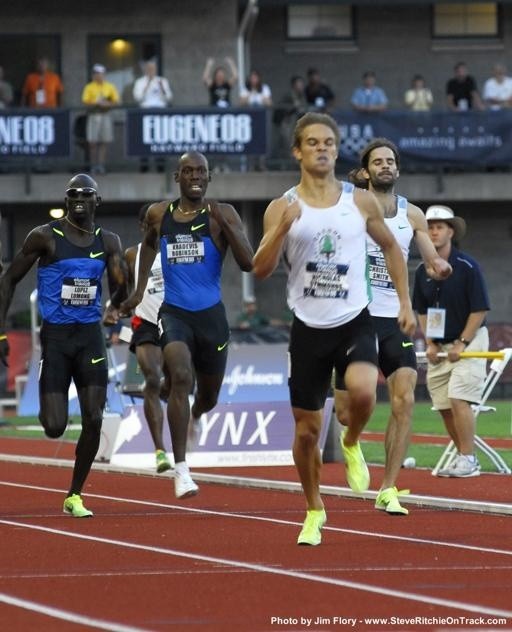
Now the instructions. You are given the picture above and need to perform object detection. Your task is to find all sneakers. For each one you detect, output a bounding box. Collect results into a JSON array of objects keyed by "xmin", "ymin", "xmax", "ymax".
[
  {"xmin": 63, "ymin": 493, "xmax": 93, "ymax": 518},
  {"xmin": 298, "ymin": 509, "xmax": 327, "ymax": 547},
  {"xmin": 156, "ymin": 449, "xmax": 171, "ymax": 473},
  {"xmin": 340, "ymin": 426, "xmax": 370, "ymax": 494},
  {"xmin": 375, "ymin": 488, "xmax": 409, "ymax": 515},
  {"xmin": 437, "ymin": 453, "xmax": 482, "ymax": 477},
  {"xmin": 175, "ymin": 468, "xmax": 199, "ymax": 499},
  {"xmin": 450, "ymin": 455, "xmax": 480, "ymax": 477}
]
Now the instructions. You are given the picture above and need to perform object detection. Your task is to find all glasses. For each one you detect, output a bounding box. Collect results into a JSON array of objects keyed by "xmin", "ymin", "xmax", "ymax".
[{"xmin": 67, "ymin": 188, "xmax": 99, "ymax": 198}]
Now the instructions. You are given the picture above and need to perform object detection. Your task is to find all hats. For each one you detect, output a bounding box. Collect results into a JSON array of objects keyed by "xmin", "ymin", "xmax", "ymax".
[
  {"xmin": 425, "ymin": 205, "xmax": 466, "ymax": 244},
  {"xmin": 93, "ymin": 64, "xmax": 105, "ymax": 72}
]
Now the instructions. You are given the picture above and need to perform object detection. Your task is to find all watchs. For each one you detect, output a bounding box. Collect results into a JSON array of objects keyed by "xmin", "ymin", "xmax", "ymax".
[{"xmin": 457, "ymin": 335, "xmax": 471, "ymax": 345}]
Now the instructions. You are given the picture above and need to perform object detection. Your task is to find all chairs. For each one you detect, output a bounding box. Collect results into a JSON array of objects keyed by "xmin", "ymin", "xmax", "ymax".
[{"xmin": 432, "ymin": 347, "xmax": 512, "ymax": 478}]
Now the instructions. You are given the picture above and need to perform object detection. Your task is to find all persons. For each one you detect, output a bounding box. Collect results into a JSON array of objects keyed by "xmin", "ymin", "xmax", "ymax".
[
  {"xmin": 330, "ymin": 137, "xmax": 456, "ymax": 516},
  {"xmin": 121, "ymin": 151, "xmax": 253, "ymax": 498},
  {"xmin": 445, "ymin": 62, "xmax": 484, "ymax": 111},
  {"xmin": 202, "ymin": 56, "xmax": 238, "ymax": 176},
  {"xmin": 1, "ymin": 70, "xmax": 14, "ymax": 111},
  {"xmin": 305, "ymin": 66, "xmax": 334, "ymax": 111},
  {"xmin": 3, "ymin": 174, "xmax": 132, "ymax": 517},
  {"xmin": 280, "ymin": 77, "xmax": 303, "ymax": 148},
  {"xmin": 412, "ymin": 206, "xmax": 490, "ymax": 480},
  {"xmin": 132, "ymin": 60, "xmax": 169, "ymax": 173},
  {"xmin": 236, "ymin": 72, "xmax": 273, "ymax": 172},
  {"xmin": 350, "ymin": 70, "xmax": 390, "ymax": 113},
  {"xmin": 106, "ymin": 201, "xmax": 196, "ymax": 473},
  {"xmin": 81, "ymin": 65, "xmax": 120, "ymax": 174},
  {"xmin": 20, "ymin": 54, "xmax": 67, "ymax": 174},
  {"xmin": 254, "ymin": 112, "xmax": 416, "ymax": 547},
  {"xmin": 484, "ymin": 61, "xmax": 511, "ymax": 111},
  {"xmin": 106, "ymin": 319, "xmax": 124, "ymax": 344},
  {"xmin": 404, "ymin": 76, "xmax": 433, "ymax": 114},
  {"xmin": 236, "ymin": 294, "xmax": 285, "ymax": 330}
]
